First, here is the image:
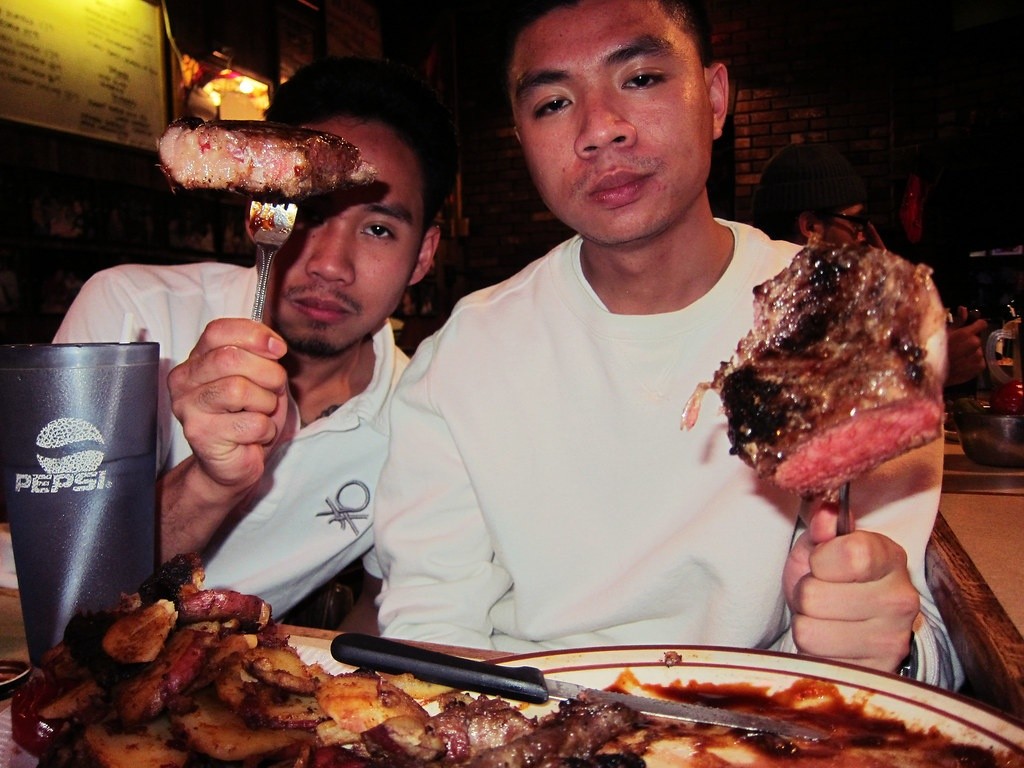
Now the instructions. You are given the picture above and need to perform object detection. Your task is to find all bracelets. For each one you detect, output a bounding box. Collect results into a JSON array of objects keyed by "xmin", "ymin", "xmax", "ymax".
[{"xmin": 896, "ymin": 630, "xmax": 918, "ymax": 679}]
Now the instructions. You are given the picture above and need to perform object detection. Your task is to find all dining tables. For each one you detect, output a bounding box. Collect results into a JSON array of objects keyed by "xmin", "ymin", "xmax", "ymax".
[{"xmin": 2, "ymin": 590, "xmax": 519, "ymax": 767}]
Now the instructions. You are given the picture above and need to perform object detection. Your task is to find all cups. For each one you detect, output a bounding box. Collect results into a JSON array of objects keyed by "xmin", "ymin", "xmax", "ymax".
[{"xmin": 0, "ymin": 338, "xmax": 161, "ymax": 670}]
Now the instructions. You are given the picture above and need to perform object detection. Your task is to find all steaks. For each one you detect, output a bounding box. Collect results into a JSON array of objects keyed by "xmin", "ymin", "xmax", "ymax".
[
  {"xmin": 10, "ymin": 549, "xmax": 649, "ymax": 768},
  {"xmin": 682, "ymin": 230, "xmax": 950, "ymax": 498},
  {"xmin": 155, "ymin": 115, "xmax": 380, "ymax": 197}
]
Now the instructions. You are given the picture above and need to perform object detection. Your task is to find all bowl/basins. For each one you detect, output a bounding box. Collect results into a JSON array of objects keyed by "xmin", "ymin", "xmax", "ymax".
[{"xmin": 953, "ymin": 407, "xmax": 1024, "ymax": 467}]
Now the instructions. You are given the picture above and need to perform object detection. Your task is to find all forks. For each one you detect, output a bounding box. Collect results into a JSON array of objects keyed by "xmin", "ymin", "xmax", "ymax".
[{"xmin": 242, "ymin": 194, "xmax": 300, "ymax": 323}]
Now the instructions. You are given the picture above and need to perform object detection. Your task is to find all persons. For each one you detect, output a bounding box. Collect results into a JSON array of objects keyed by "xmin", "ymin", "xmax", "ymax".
[
  {"xmin": 0, "ymin": 52, "xmax": 451, "ymax": 621},
  {"xmin": 749, "ymin": 143, "xmax": 988, "ymax": 389},
  {"xmin": 373, "ymin": 1, "xmax": 968, "ymax": 696}
]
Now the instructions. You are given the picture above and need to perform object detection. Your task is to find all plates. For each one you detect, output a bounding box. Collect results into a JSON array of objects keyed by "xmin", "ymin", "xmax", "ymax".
[{"xmin": 339, "ymin": 645, "xmax": 1024, "ymax": 767}]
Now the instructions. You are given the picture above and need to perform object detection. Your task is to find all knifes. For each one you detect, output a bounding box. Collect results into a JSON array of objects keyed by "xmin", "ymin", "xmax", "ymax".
[{"xmin": 329, "ymin": 626, "xmax": 834, "ymax": 743}]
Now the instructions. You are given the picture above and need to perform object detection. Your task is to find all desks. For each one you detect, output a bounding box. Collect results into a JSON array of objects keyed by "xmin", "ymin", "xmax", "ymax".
[{"xmin": 936, "ymin": 489, "xmax": 1023, "ymax": 723}]
